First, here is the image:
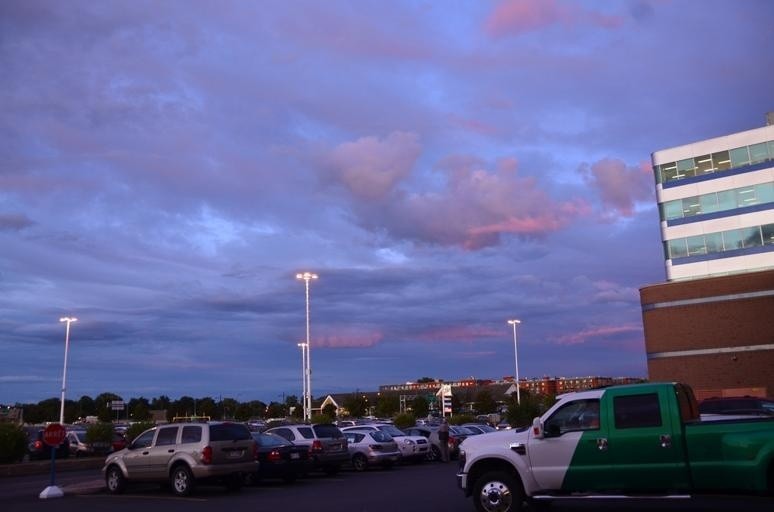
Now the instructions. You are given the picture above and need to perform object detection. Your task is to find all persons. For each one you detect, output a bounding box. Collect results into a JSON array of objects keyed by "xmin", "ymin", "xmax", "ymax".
[{"xmin": 437, "ymin": 420, "xmax": 449, "ymax": 463}]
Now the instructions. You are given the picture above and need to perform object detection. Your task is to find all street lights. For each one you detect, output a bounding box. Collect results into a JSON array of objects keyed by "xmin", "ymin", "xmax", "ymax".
[
  {"xmin": 57, "ymin": 317, "xmax": 79, "ymax": 426},
  {"xmin": 294, "ymin": 270, "xmax": 319, "ymax": 422},
  {"xmin": 506, "ymin": 317, "xmax": 524, "ymax": 407}
]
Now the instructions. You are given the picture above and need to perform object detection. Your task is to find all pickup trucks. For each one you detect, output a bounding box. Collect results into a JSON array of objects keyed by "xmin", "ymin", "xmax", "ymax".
[{"xmin": 454, "ymin": 381, "xmax": 773, "ymax": 512}]
